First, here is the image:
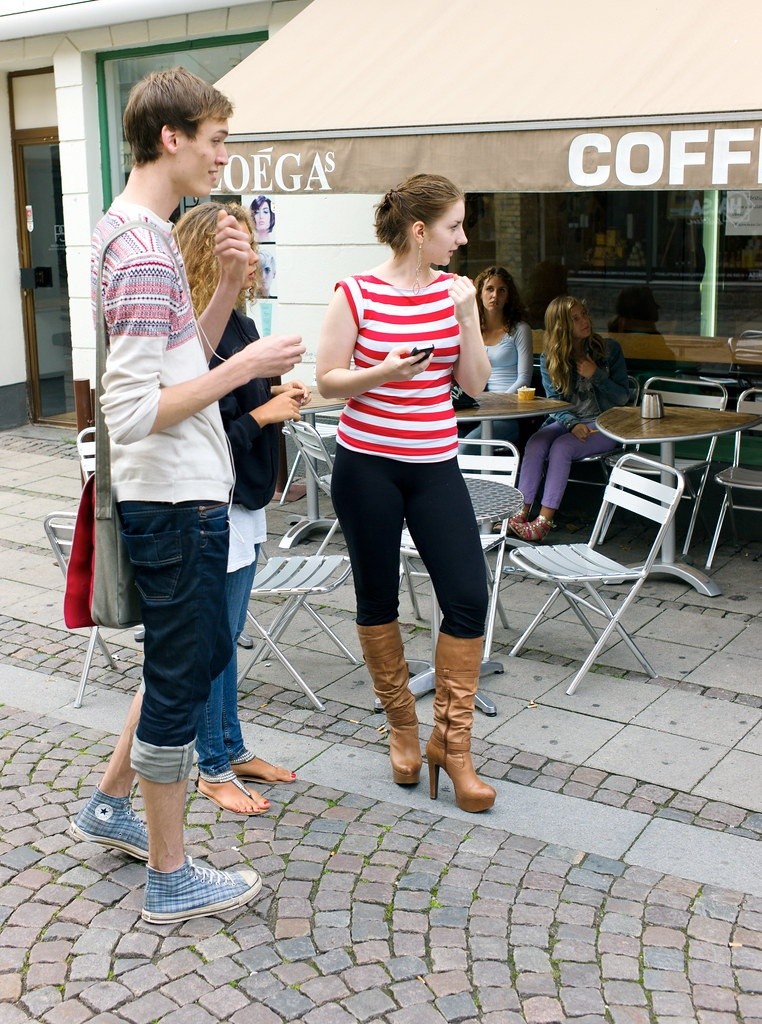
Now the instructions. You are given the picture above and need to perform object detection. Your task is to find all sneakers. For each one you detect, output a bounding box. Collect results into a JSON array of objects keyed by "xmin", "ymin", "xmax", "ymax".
[
  {"xmin": 143, "ymin": 854, "xmax": 262, "ymax": 924},
  {"xmin": 69, "ymin": 783, "xmax": 150, "ymax": 862}
]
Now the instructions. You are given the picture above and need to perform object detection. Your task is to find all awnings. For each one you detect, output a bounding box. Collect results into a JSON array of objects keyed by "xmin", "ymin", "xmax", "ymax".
[{"xmin": 207, "ymin": 0, "xmax": 762, "ymax": 194}]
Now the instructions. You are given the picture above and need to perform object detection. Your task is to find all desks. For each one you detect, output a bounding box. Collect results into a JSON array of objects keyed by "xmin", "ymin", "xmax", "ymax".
[
  {"xmin": 374, "ymin": 478, "xmax": 525, "ymax": 716},
  {"xmin": 455, "ymin": 392, "xmax": 575, "ymax": 547},
  {"xmin": 595, "ymin": 405, "xmax": 762, "ymax": 596},
  {"xmin": 277, "ymin": 387, "xmax": 350, "ymax": 549}
]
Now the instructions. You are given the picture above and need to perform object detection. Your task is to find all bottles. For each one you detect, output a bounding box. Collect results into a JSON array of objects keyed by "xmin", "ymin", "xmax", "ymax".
[
  {"xmin": 719, "ymin": 236, "xmax": 761, "ymax": 268},
  {"xmin": 580, "ymin": 213, "xmax": 589, "ymax": 228}
]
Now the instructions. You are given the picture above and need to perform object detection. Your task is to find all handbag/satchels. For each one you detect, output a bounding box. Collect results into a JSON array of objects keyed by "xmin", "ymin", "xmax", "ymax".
[
  {"xmin": 450, "ymin": 375, "xmax": 480, "ymax": 411},
  {"xmin": 65, "ymin": 475, "xmax": 141, "ymax": 629}
]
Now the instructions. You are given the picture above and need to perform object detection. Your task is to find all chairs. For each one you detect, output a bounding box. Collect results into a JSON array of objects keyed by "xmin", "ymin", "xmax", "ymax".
[{"xmin": 44, "ymin": 364, "xmax": 762, "ymax": 711}]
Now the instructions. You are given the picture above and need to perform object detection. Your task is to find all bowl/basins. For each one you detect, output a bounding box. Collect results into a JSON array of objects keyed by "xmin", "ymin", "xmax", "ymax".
[{"xmin": 517, "ymin": 388, "xmax": 536, "ymax": 400}]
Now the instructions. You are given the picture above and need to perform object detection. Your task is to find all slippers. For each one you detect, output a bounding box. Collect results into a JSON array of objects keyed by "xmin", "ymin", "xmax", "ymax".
[
  {"xmin": 197, "ymin": 787, "xmax": 270, "ymax": 815},
  {"xmin": 236, "ymin": 758, "xmax": 293, "ymax": 784}
]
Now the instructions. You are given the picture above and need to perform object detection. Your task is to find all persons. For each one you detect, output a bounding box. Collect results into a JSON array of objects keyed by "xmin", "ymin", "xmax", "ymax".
[
  {"xmin": 259, "ymin": 248, "xmax": 276, "ymax": 296},
  {"xmin": 455, "ymin": 265, "xmax": 537, "ymax": 476},
  {"xmin": 172, "ymin": 199, "xmax": 312, "ymax": 817},
  {"xmin": 317, "ymin": 172, "xmax": 500, "ymax": 815},
  {"xmin": 494, "ymin": 295, "xmax": 631, "ymax": 544},
  {"xmin": 73, "ymin": 63, "xmax": 309, "ymax": 929},
  {"xmin": 248, "ymin": 195, "xmax": 275, "ymax": 242}
]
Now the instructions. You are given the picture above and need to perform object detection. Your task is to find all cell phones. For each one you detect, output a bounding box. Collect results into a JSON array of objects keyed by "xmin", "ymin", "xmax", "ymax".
[{"xmin": 410, "ymin": 344, "xmax": 434, "ymax": 366}]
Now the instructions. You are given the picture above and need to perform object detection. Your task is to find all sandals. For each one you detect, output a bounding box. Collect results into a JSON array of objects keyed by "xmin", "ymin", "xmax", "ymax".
[
  {"xmin": 493, "ymin": 508, "xmax": 530, "ymax": 536},
  {"xmin": 509, "ymin": 515, "xmax": 556, "ymax": 544}
]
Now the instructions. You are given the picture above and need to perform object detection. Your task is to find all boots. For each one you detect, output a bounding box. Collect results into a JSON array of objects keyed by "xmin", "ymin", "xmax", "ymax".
[
  {"xmin": 356, "ymin": 617, "xmax": 422, "ymax": 783},
  {"xmin": 425, "ymin": 634, "xmax": 496, "ymax": 812}
]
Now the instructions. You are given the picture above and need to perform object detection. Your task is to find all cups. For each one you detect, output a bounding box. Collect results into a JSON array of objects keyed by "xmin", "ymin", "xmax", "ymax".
[
  {"xmin": 641, "ymin": 393, "xmax": 662, "ymax": 419},
  {"xmin": 568, "ymin": 223, "xmax": 579, "ymax": 229}
]
